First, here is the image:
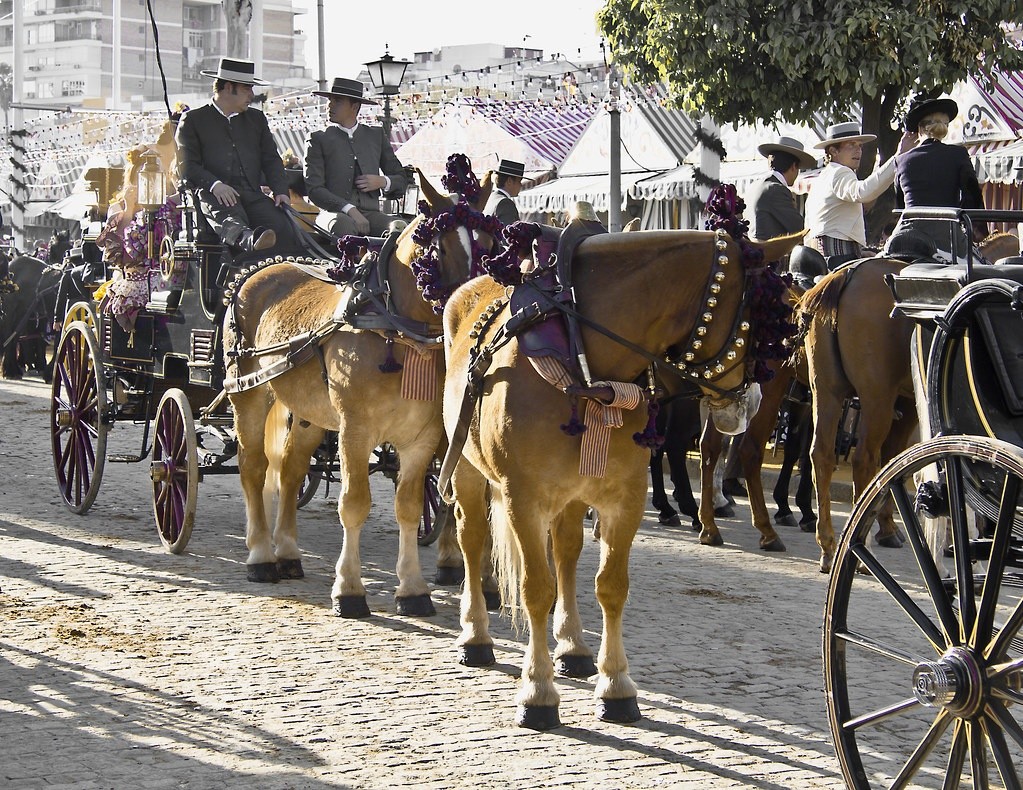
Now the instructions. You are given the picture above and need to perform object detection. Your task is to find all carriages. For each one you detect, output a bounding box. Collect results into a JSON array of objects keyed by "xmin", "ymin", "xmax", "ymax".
[{"xmin": 47, "ymin": 193, "xmax": 812, "ymax": 733}]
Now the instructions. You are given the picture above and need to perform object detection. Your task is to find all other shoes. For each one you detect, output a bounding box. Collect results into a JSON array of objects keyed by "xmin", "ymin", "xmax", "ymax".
[{"xmin": 253, "ymin": 228, "xmax": 277, "ymax": 251}]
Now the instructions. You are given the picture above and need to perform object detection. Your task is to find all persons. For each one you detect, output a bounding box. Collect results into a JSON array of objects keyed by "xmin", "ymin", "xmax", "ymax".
[
  {"xmin": 303, "ymin": 77, "xmax": 407, "ymax": 237},
  {"xmin": 172, "ymin": 58, "xmax": 295, "ymax": 253},
  {"xmin": 49, "ymin": 228, "xmax": 73, "ymax": 264},
  {"xmin": 483, "ymin": 159, "xmax": 535, "ymax": 225},
  {"xmin": 889, "ymin": 99, "xmax": 989, "ymax": 258},
  {"xmin": 748, "ymin": 136, "xmax": 816, "ymax": 242},
  {"xmin": 803, "ymin": 122, "xmax": 920, "ymax": 259}
]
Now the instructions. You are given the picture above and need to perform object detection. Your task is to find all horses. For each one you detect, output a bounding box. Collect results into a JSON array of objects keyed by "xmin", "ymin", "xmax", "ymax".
[
  {"xmin": 442, "ymin": 230, "xmax": 763, "ymax": 733},
  {"xmin": 0, "ymin": 229, "xmax": 76, "ymax": 384},
  {"xmin": 804, "ymin": 231, "xmax": 1019, "ymax": 573},
  {"xmin": 647, "ymin": 273, "xmax": 862, "ymax": 532},
  {"xmin": 222, "ymin": 167, "xmax": 508, "ymax": 620}
]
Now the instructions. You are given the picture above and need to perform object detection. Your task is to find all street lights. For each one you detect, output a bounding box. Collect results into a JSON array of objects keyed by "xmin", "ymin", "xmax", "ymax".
[{"xmin": 361, "ymin": 42, "xmax": 415, "ymax": 215}]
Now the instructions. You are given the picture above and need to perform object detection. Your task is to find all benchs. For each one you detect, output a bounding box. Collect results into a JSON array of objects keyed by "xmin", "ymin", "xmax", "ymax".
[
  {"xmin": 173, "ymin": 167, "xmax": 342, "ymax": 261},
  {"xmin": 882, "ymin": 205, "xmax": 1023, "ymax": 319}
]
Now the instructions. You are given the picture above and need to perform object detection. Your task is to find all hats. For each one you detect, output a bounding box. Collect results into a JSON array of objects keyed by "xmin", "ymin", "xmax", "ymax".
[
  {"xmin": 200, "ymin": 59, "xmax": 272, "ymax": 87},
  {"xmin": 488, "ymin": 159, "xmax": 536, "ymax": 182},
  {"xmin": 312, "ymin": 78, "xmax": 379, "ymax": 105},
  {"xmin": 758, "ymin": 136, "xmax": 816, "ymax": 170},
  {"xmin": 905, "ymin": 99, "xmax": 959, "ymax": 133},
  {"xmin": 813, "ymin": 122, "xmax": 878, "ymax": 149}
]
